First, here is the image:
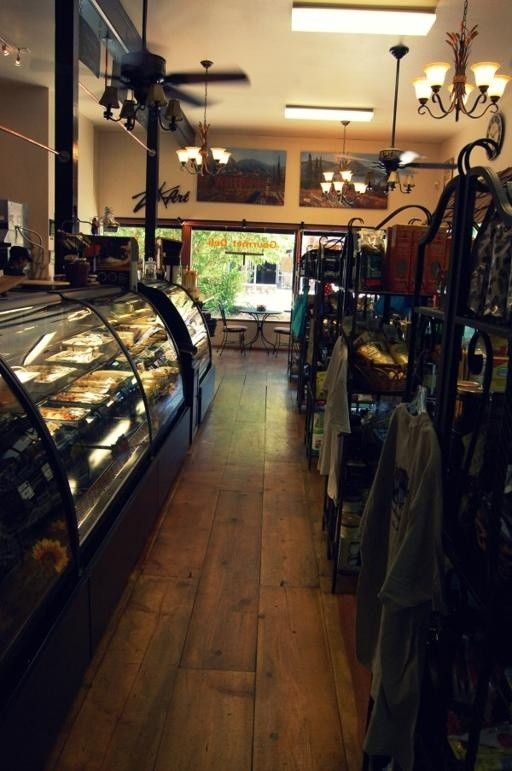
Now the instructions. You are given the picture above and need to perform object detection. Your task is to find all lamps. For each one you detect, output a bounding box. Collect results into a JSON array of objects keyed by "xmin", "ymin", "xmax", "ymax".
[
  {"xmin": 318, "ymin": 121, "xmax": 368, "ymax": 202},
  {"xmin": 99, "ymin": 81, "xmax": 184, "ymax": 133},
  {"xmin": 287, "ymin": 5, "xmax": 438, "ymax": 38},
  {"xmin": 0, "ymin": 33, "xmax": 28, "ymax": 67},
  {"xmin": 174, "ymin": 60, "xmax": 232, "ymax": 177},
  {"xmin": 411, "ymin": 0, "xmax": 512, "ymax": 122},
  {"xmin": 282, "ymin": 105, "xmax": 373, "ymax": 123}
]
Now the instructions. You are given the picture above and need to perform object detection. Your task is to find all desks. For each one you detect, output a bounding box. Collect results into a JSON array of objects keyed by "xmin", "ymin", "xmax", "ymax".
[{"xmin": 237, "ymin": 308, "xmax": 282, "ymax": 356}]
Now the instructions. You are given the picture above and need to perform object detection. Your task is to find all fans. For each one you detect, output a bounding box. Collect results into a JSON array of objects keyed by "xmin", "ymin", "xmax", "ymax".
[
  {"xmin": 30, "ymin": 0, "xmax": 252, "ymax": 108},
  {"xmin": 336, "ymin": 44, "xmax": 457, "ymax": 171}
]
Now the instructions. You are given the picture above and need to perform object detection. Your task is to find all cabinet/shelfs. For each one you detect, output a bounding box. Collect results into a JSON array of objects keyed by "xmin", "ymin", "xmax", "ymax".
[
  {"xmin": 0, "ymin": 277, "xmax": 215, "ymax": 770},
  {"xmin": 287, "ymin": 139, "xmax": 512, "ymax": 770}
]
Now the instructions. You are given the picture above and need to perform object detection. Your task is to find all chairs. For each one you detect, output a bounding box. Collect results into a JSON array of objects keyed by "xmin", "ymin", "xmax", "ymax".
[
  {"xmin": 273, "ymin": 326, "xmax": 292, "ymax": 358},
  {"xmin": 217, "ymin": 305, "xmax": 248, "ymax": 357}
]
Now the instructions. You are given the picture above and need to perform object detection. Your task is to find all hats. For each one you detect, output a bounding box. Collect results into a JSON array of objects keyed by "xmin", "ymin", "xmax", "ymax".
[{"xmin": 9, "ymin": 246, "xmax": 32, "ymax": 262}]
